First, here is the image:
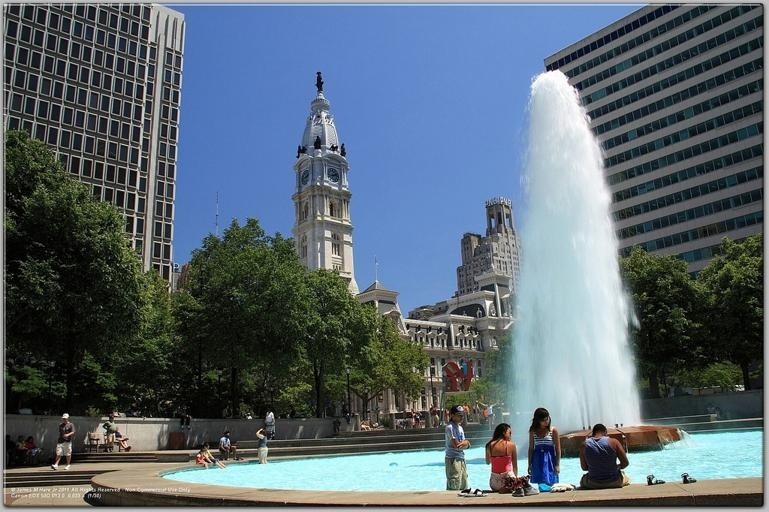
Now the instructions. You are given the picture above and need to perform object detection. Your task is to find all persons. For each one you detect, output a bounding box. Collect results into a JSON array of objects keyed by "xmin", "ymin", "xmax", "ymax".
[
  {"xmin": 332, "ymin": 399, "xmax": 501, "ymax": 435},
  {"xmin": 6, "ymin": 413, "xmax": 132, "ymax": 470},
  {"xmin": 444, "ymin": 405, "xmax": 472, "ymax": 490},
  {"xmin": 196, "ymin": 408, "xmax": 276, "ymax": 470},
  {"xmin": 527, "ymin": 408, "xmax": 562, "ymax": 485},
  {"xmin": 485, "ymin": 423, "xmax": 518, "ymax": 491},
  {"xmin": 579, "ymin": 424, "xmax": 630, "ymax": 489}
]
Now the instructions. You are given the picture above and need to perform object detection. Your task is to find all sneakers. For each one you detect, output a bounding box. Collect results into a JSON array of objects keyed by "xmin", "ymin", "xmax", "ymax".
[
  {"xmin": 524, "ymin": 487, "xmax": 539, "ymax": 495},
  {"xmin": 64, "ymin": 466, "xmax": 71, "ymax": 469},
  {"xmin": 51, "ymin": 464, "xmax": 58, "ymax": 470},
  {"xmin": 512, "ymin": 488, "xmax": 524, "ymax": 497}
]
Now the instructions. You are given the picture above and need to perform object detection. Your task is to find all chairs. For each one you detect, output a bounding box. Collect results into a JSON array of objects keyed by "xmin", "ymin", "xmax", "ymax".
[
  {"xmin": 88, "ymin": 432, "xmax": 111, "ymax": 453},
  {"xmin": 104, "ymin": 433, "xmax": 121, "ymax": 453}
]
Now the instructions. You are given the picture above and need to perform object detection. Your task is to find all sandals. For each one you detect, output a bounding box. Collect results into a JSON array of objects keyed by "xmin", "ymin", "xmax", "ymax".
[
  {"xmin": 681, "ymin": 473, "xmax": 696, "ymax": 483},
  {"xmin": 647, "ymin": 475, "xmax": 665, "ymax": 485}
]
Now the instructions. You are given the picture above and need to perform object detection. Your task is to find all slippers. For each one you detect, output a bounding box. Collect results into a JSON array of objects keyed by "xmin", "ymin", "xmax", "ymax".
[
  {"xmin": 458, "ymin": 488, "xmax": 474, "ymax": 495},
  {"xmin": 465, "ymin": 490, "xmax": 488, "ymax": 496}
]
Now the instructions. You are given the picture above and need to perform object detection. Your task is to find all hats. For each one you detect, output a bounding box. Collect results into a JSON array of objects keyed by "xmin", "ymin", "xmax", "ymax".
[
  {"xmin": 61, "ymin": 413, "xmax": 69, "ymax": 420},
  {"xmin": 451, "ymin": 405, "xmax": 465, "ymax": 416}
]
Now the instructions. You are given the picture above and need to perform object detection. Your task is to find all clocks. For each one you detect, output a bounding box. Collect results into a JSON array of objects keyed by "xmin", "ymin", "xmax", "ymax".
[
  {"xmin": 301, "ymin": 169, "xmax": 309, "ymax": 185},
  {"xmin": 328, "ymin": 168, "xmax": 339, "ymax": 182}
]
